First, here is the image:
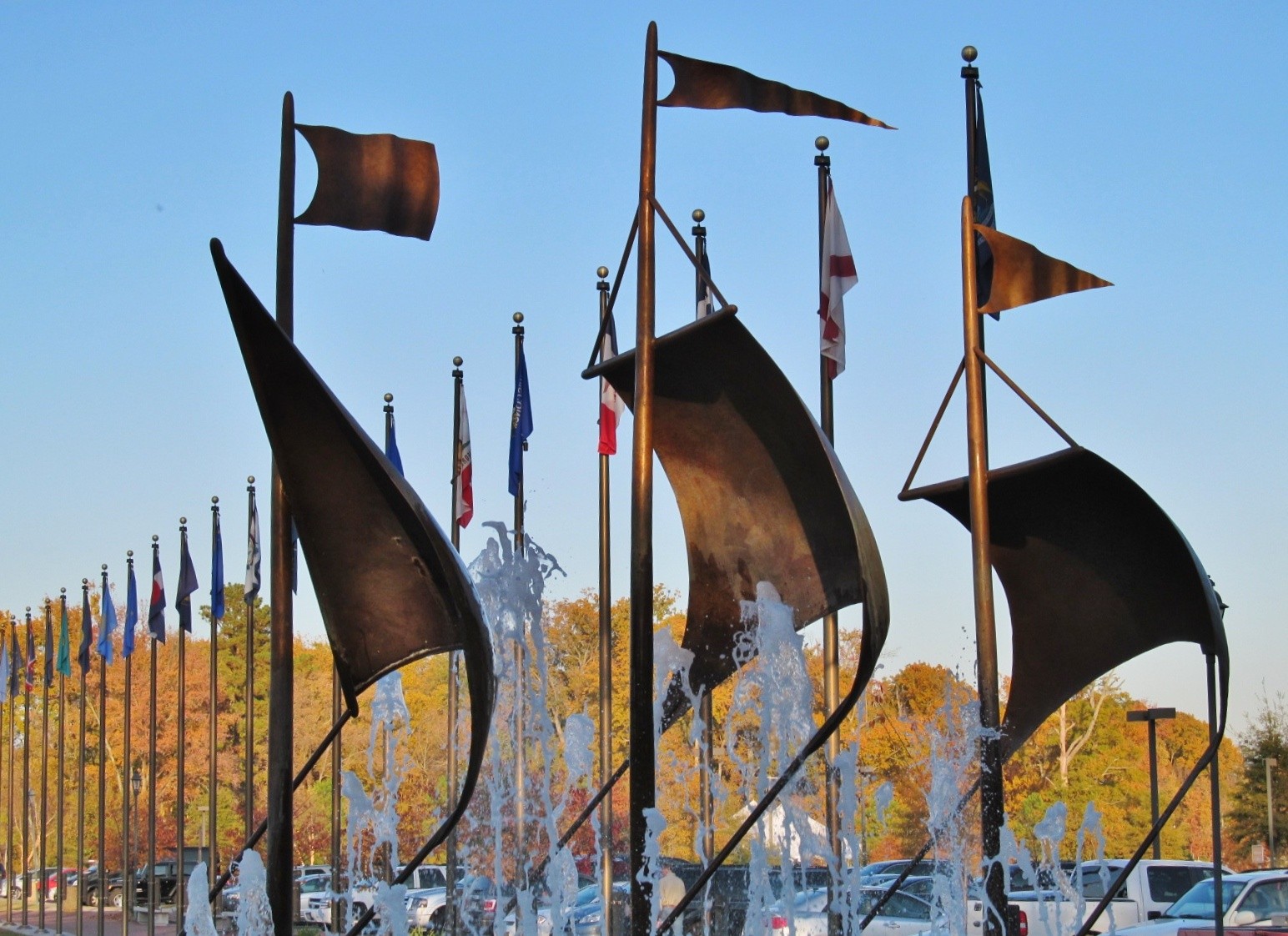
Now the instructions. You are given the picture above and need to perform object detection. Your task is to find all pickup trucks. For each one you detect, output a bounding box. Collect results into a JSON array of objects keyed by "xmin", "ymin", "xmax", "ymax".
[{"xmin": 964, "ymin": 859, "xmax": 1275, "ymax": 936}]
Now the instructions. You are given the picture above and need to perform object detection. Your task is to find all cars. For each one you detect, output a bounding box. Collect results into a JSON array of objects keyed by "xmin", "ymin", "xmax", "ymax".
[
  {"xmin": 221, "ymin": 857, "xmax": 692, "ymax": 936},
  {"xmin": 0, "ymin": 863, "xmax": 141, "ymax": 907},
  {"xmin": 743, "ymin": 858, "xmax": 1076, "ymax": 936}
]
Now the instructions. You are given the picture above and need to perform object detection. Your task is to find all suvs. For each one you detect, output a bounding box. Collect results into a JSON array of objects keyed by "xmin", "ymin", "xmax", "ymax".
[
  {"xmin": 1099, "ymin": 868, "xmax": 1288, "ymax": 936},
  {"xmin": 135, "ymin": 861, "xmax": 205, "ymax": 904}
]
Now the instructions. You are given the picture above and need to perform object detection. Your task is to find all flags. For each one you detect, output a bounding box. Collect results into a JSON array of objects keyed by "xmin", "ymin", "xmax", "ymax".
[
  {"xmin": 597, "ymin": 312, "xmax": 623, "ymax": 454},
  {"xmin": 976, "ymin": 81, "xmax": 1001, "ymax": 322},
  {"xmin": 175, "ymin": 537, "xmax": 198, "ymax": 633},
  {"xmin": 244, "ymin": 496, "xmax": 261, "ymax": 605},
  {"xmin": 148, "ymin": 551, "xmax": 166, "ymax": 646},
  {"xmin": 456, "ymin": 386, "xmax": 474, "ymax": 528},
  {"xmin": 25, "ymin": 622, "xmax": 36, "ymax": 690},
  {"xmin": 56, "ymin": 609, "xmax": 71, "ymax": 677},
  {"xmin": 0, "ymin": 644, "xmax": 10, "ymax": 702},
  {"xmin": 122, "ymin": 567, "xmax": 138, "ymax": 657},
  {"xmin": 211, "ymin": 518, "xmax": 224, "ymax": 619},
  {"xmin": 47, "ymin": 619, "xmax": 53, "ymax": 685},
  {"xmin": 11, "ymin": 636, "xmax": 23, "ymax": 697},
  {"xmin": 78, "ymin": 593, "xmax": 93, "ymax": 674},
  {"xmin": 821, "ymin": 177, "xmax": 859, "ymax": 379},
  {"xmin": 508, "ymin": 348, "xmax": 534, "ymax": 495},
  {"xmin": 698, "ymin": 250, "xmax": 714, "ymax": 320},
  {"xmin": 389, "ymin": 416, "xmax": 404, "ymax": 475},
  {"xmin": 98, "ymin": 584, "xmax": 117, "ymax": 665},
  {"xmin": 292, "ymin": 521, "xmax": 298, "ymax": 594}
]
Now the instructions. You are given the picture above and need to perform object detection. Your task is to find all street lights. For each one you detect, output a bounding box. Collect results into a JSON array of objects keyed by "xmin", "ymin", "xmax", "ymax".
[
  {"xmin": 1126, "ymin": 707, "xmax": 1176, "ymax": 877},
  {"xmin": 197, "ymin": 806, "xmax": 208, "ymax": 846},
  {"xmin": 131, "ymin": 771, "xmax": 141, "ymax": 906},
  {"xmin": 1260, "ymin": 757, "xmax": 1277, "ymax": 869}
]
